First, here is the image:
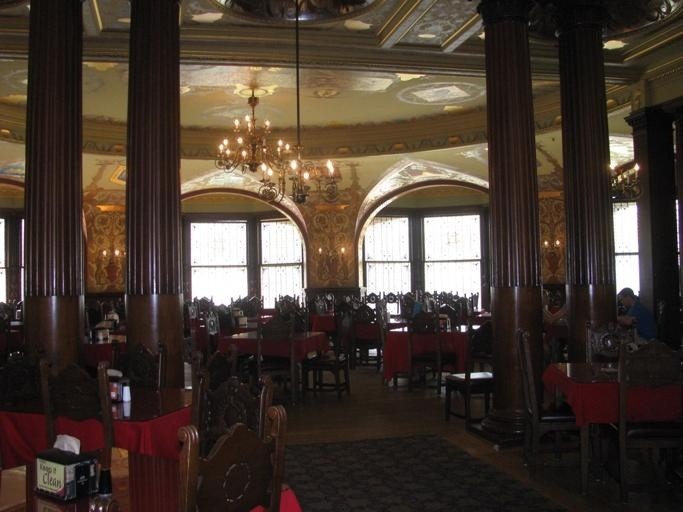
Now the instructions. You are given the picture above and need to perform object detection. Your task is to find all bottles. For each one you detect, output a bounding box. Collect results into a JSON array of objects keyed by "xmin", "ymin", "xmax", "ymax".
[
  {"xmin": 89, "ymin": 497, "xmax": 114, "ymax": 512},
  {"xmin": 107, "ymin": 376, "xmax": 131, "ymax": 402},
  {"xmin": 111, "ymin": 403, "xmax": 131, "ymax": 420},
  {"xmin": 92, "ymin": 457, "xmax": 114, "ymax": 497}
]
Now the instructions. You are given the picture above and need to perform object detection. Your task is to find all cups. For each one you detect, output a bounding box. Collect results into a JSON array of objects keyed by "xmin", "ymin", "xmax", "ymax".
[{"xmin": 439, "ymin": 314, "xmax": 450, "ymax": 330}]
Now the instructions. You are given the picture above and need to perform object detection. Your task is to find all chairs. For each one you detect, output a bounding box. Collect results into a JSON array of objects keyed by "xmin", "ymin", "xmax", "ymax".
[
  {"xmin": 514, "ymin": 326, "xmax": 598, "ymax": 476},
  {"xmin": 592, "ymin": 342, "xmax": 679, "ymax": 508},
  {"xmin": 310, "ymin": 291, "xmax": 679, "ymax": 399},
  {"xmin": 0, "ymin": 276, "xmax": 308, "ymax": 510},
  {"xmin": 444, "ymin": 321, "xmax": 499, "ymax": 432}
]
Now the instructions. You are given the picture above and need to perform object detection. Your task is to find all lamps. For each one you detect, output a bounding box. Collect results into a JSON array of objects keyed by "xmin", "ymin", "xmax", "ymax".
[
  {"xmin": 608, "ymin": 161, "xmax": 645, "ymax": 203},
  {"xmin": 211, "ymin": 94, "xmax": 295, "ymax": 176},
  {"xmin": 256, "ymin": 0, "xmax": 343, "ymax": 205}
]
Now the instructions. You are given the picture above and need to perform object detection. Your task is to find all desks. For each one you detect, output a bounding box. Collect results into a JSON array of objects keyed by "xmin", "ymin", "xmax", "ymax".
[{"xmin": 543, "ymin": 361, "xmax": 679, "ymax": 497}]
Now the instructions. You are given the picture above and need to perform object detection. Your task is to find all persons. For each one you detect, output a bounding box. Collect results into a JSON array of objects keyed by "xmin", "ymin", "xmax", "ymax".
[
  {"xmin": 617, "ymin": 288, "xmax": 657, "ymax": 346},
  {"xmin": 542, "ymin": 288, "xmax": 567, "ymax": 363}
]
